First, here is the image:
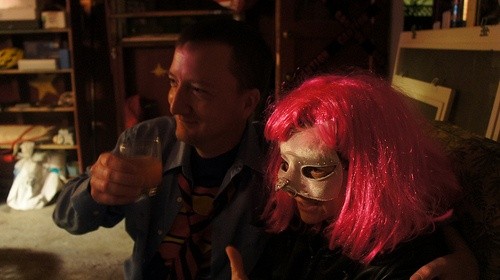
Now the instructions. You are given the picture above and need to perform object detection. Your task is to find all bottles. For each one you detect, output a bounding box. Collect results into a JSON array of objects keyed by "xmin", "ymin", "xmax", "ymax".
[{"xmin": 449, "ymin": 0, "xmax": 464, "ymax": 29}]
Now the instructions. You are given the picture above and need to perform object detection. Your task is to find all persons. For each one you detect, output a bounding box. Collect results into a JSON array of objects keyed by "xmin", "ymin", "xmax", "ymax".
[{"xmin": 52, "ymin": 15, "xmax": 491, "ymax": 280}]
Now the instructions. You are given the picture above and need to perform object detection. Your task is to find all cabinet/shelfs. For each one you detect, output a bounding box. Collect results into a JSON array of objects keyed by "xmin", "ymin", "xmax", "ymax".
[
  {"xmin": 105, "ymin": 0, "xmax": 393, "ymax": 145},
  {"xmin": 0, "ymin": 0, "xmax": 116, "ymax": 175}
]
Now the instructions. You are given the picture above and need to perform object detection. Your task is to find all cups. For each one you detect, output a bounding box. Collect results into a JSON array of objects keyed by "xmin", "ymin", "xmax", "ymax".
[{"xmin": 113, "ymin": 134, "xmax": 162, "ymax": 198}]
